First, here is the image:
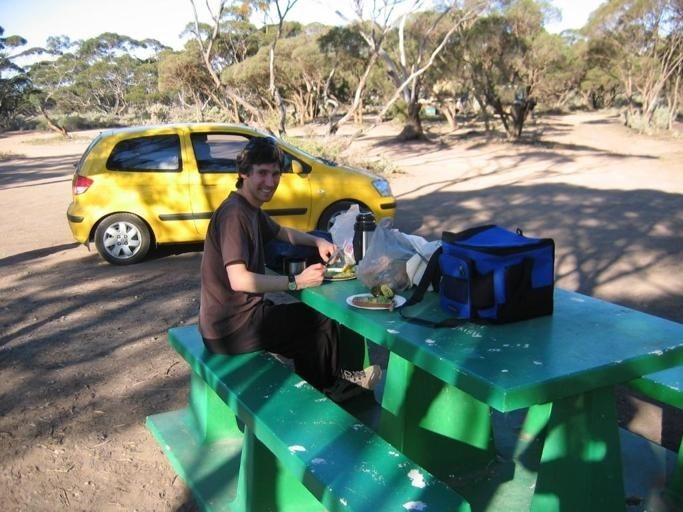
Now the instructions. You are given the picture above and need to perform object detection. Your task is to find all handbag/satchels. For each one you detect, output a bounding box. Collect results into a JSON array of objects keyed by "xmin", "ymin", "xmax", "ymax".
[{"xmin": 393, "ymin": 223, "xmax": 559, "ymax": 333}]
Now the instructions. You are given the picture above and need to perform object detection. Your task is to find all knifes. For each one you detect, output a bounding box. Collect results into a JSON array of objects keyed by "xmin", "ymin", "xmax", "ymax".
[{"xmin": 323, "ymin": 249, "xmax": 341, "ymax": 267}]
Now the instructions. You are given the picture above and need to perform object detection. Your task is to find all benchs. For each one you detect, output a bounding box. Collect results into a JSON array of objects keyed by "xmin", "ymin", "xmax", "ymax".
[
  {"xmin": 144, "ymin": 324, "xmax": 472, "ymax": 512},
  {"xmin": 520, "ymin": 364, "xmax": 683, "ymax": 499}
]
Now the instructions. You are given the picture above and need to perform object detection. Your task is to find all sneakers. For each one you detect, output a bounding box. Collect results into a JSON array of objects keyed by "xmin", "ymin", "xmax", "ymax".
[{"xmin": 322, "ymin": 362, "xmax": 384, "ymax": 406}]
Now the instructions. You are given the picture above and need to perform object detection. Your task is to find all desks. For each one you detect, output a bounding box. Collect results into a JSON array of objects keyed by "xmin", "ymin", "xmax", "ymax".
[{"xmin": 285, "ymin": 269, "xmax": 683, "ymax": 512}]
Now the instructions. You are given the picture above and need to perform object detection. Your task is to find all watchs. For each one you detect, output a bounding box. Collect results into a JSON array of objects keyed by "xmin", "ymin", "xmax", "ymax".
[{"xmin": 287, "ymin": 274, "xmax": 296, "ymax": 295}]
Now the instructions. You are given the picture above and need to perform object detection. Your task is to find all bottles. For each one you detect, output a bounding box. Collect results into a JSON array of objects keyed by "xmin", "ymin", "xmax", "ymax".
[
  {"xmin": 353, "ymin": 214, "xmax": 377, "ymax": 266},
  {"xmin": 284, "ymin": 256, "xmax": 307, "ymax": 276}
]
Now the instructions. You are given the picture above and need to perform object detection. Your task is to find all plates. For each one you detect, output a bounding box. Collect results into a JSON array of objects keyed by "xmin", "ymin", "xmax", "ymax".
[
  {"xmin": 323, "ymin": 268, "xmax": 357, "ymax": 282},
  {"xmin": 346, "ymin": 293, "xmax": 407, "ymax": 310}
]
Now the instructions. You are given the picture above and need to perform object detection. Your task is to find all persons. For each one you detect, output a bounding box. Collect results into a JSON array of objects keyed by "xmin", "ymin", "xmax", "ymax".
[{"xmin": 197, "ymin": 137, "xmax": 382, "ymax": 404}]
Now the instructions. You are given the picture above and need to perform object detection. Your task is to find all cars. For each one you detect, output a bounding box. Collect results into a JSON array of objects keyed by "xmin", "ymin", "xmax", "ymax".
[{"xmin": 67, "ymin": 123, "xmax": 397, "ymax": 266}]
